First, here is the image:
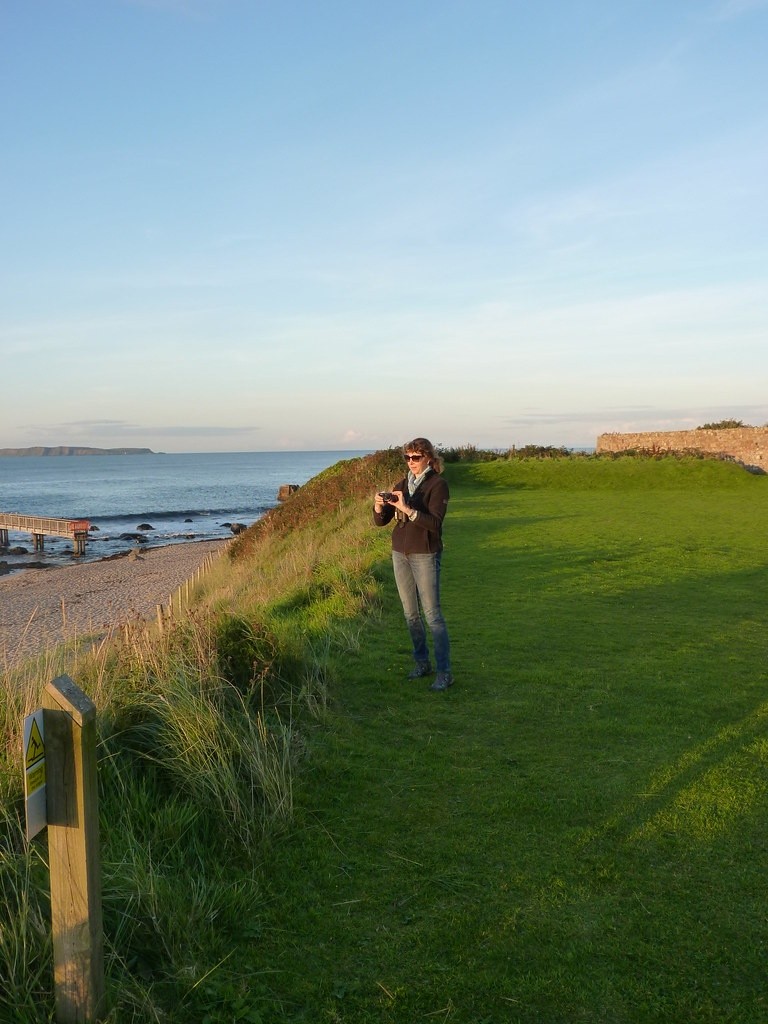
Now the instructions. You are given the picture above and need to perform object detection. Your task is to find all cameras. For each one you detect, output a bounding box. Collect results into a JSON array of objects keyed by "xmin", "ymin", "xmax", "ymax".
[{"xmin": 380, "ymin": 493, "xmax": 398, "ymax": 502}]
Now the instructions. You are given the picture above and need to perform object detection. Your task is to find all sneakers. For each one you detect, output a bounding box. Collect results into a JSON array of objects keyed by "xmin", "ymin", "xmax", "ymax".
[
  {"xmin": 408, "ymin": 661, "xmax": 432, "ymax": 678},
  {"xmin": 430, "ymin": 672, "xmax": 454, "ymax": 690}
]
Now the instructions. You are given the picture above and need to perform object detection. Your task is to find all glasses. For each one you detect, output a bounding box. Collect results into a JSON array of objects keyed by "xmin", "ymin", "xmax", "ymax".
[{"xmin": 404, "ymin": 455, "xmax": 426, "ymax": 462}]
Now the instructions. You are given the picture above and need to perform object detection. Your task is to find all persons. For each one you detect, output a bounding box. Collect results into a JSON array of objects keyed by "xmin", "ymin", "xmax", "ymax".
[{"xmin": 372, "ymin": 438, "xmax": 455, "ymax": 691}]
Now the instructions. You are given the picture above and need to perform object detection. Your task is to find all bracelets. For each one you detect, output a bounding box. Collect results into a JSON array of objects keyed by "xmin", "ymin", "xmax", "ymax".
[{"xmin": 408, "ymin": 509, "xmax": 415, "ymax": 517}]
